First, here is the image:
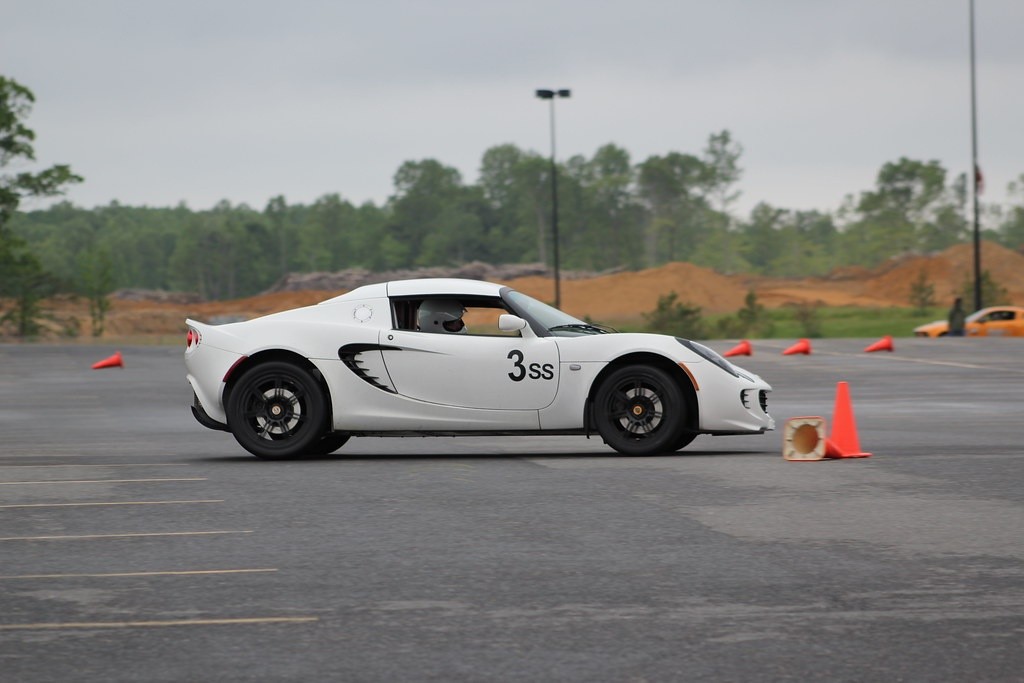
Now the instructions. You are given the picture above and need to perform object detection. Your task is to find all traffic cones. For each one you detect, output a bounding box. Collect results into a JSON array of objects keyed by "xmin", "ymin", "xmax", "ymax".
[
  {"xmin": 91, "ymin": 351, "xmax": 125, "ymax": 369},
  {"xmin": 780, "ymin": 416, "xmax": 843, "ymax": 461},
  {"xmin": 782, "ymin": 339, "xmax": 811, "ymax": 356},
  {"xmin": 863, "ymin": 334, "xmax": 896, "ymax": 353},
  {"xmin": 721, "ymin": 339, "xmax": 752, "ymax": 357},
  {"xmin": 826, "ymin": 382, "xmax": 873, "ymax": 459}
]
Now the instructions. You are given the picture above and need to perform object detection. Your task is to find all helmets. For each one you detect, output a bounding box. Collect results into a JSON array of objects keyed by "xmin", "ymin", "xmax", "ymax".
[{"xmin": 415, "ymin": 298, "xmax": 468, "ymax": 335}]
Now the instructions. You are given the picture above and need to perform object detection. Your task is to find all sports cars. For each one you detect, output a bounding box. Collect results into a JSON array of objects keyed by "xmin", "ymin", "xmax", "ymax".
[{"xmin": 183, "ymin": 277, "xmax": 776, "ymax": 458}]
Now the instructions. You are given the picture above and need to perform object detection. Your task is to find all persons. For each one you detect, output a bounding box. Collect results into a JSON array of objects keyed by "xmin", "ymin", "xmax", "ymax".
[
  {"xmin": 417, "ymin": 298, "xmax": 468, "ymax": 335},
  {"xmin": 948, "ymin": 298, "xmax": 965, "ymax": 337}
]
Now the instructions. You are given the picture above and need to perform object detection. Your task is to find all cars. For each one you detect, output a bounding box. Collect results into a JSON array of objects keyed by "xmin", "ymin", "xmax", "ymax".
[{"xmin": 912, "ymin": 305, "xmax": 1024, "ymax": 337}]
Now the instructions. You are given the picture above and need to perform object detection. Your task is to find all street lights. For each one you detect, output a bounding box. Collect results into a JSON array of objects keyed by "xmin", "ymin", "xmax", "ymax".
[{"xmin": 535, "ymin": 87, "xmax": 572, "ymax": 307}]
